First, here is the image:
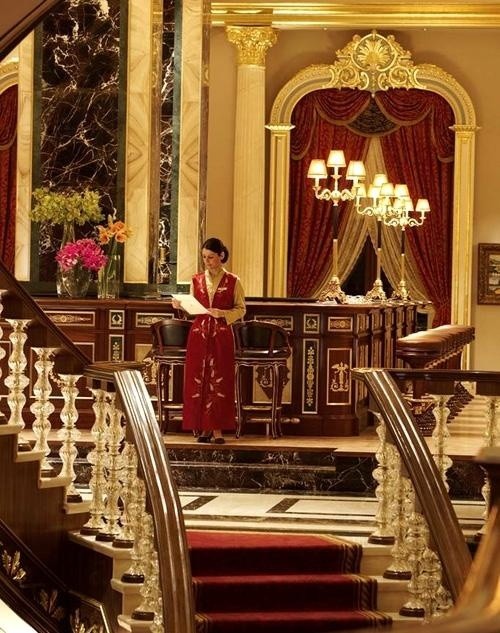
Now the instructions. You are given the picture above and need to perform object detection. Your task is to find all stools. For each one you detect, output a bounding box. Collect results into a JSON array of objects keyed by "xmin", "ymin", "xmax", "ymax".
[{"xmin": 395, "ymin": 323, "xmax": 475, "ymax": 437}]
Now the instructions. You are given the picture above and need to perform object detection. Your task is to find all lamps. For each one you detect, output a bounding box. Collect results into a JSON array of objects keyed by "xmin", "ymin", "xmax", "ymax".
[{"xmin": 307, "ymin": 149, "xmax": 430, "ymax": 306}]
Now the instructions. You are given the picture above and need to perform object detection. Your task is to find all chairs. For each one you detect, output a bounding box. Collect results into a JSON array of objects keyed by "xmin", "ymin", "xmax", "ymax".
[
  {"xmin": 149, "ymin": 319, "xmax": 193, "ymax": 433},
  {"xmin": 231, "ymin": 321, "xmax": 292, "ymax": 440}
]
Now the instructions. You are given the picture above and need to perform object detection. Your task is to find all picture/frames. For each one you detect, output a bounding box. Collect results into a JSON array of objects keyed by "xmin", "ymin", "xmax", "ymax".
[{"xmin": 477, "ymin": 243, "xmax": 500, "ymax": 305}]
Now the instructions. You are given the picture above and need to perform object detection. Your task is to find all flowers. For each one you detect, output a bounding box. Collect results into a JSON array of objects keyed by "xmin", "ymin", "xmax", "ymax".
[
  {"xmin": 96, "ymin": 214, "xmax": 132, "ymax": 254},
  {"xmin": 55, "ymin": 239, "xmax": 109, "ymax": 271},
  {"xmin": 28, "ymin": 186, "xmax": 106, "ymax": 227}
]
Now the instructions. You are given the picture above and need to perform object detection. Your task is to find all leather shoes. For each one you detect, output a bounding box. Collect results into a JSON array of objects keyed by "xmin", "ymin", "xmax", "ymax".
[
  {"xmin": 212, "ymin": 430, "xmax": 224, "ymax": 443},
  {"xmin": 198, "ymin": 430, "xmax": 213, "ymax": 441}
]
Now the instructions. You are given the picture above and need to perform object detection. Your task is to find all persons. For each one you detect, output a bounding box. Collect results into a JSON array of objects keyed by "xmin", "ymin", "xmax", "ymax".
[{"xmin": 170, "ymin": 235, "xmax": 250, "ymax": 442}]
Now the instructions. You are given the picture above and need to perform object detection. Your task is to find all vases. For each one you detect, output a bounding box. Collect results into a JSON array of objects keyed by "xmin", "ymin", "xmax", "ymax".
[
  {"xmin": 56, "ymin": 224, "xmax": 76, "ymax": 299},
  {"xmin": 96, "ymin": 254, "xmax": 121, "ymax": 300},
  {"xmin": 61, "ymin": 269, "xmax": 91, "ymax": 298}
]
